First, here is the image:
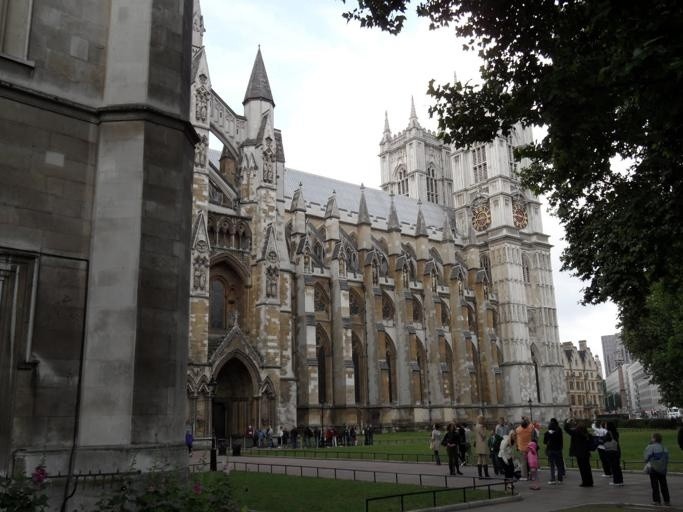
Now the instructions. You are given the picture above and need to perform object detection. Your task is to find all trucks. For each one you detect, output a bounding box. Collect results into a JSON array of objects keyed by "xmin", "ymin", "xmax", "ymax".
[{"xmin": 667, "ymin": 407, "xmax": 682, "ymax": 419}]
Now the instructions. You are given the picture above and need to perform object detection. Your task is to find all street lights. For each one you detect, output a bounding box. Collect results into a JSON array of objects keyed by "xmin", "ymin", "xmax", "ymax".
[
  {"xmin": 527, "ymin": 398, "xmax": 534, "ymax": 422},
  {"xmin": 319, "ymin": 396, "xmax": 326, "ymax": 446},
  {"xmin": 207, "ymin": 375, "xmax": 218, "ymax": 472}
]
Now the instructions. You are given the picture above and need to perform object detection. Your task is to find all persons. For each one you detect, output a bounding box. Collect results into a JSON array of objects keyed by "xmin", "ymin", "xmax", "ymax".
[
  {"xmin": 430, "ymin": 415, "xmax": 625, "ymax": 491},
  {"xmin": 253, "ymin": 423, "xmax": 374, "ymax": 449},
  {"xmin": 643, "ymin": 433, "xmax": 670, "ymax": 506},
  {"xmin": 186, "ymin": 430, "xmax": 193, "ymax": 457}
]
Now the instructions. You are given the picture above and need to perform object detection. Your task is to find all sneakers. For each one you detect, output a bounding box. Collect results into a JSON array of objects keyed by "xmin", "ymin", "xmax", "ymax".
[
  {"xmin": 449, "ymin": 470, "xmax": 624, "ymax": 490},
  {"xmin": 650, "ymin": 501, "xmax": 672, "ymax": 506}
]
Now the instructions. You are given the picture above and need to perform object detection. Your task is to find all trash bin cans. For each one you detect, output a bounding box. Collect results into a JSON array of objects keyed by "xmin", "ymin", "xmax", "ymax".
[{"xmin": 218, "ymin": 436, "xmax": 243, "ymax": 456}]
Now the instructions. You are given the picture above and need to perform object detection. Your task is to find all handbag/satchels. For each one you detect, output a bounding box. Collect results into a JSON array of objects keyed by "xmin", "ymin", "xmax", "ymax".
[{"xmin": 643, "ymin": 457, "xmax": 666, "ymax": 475}]
